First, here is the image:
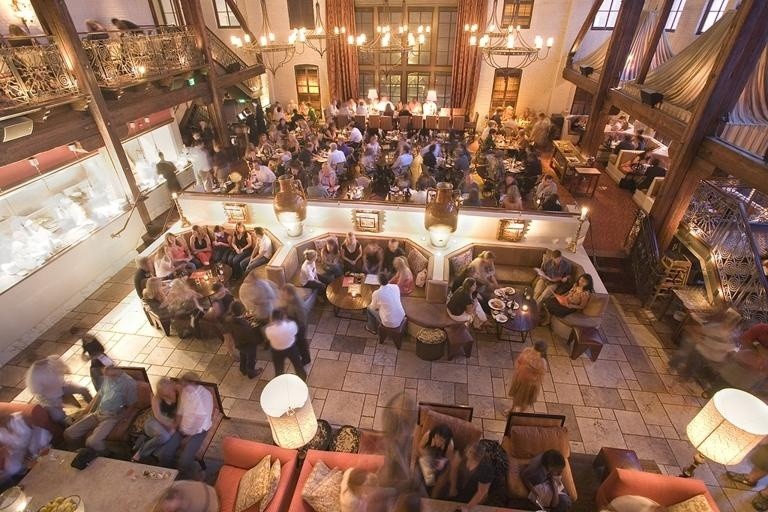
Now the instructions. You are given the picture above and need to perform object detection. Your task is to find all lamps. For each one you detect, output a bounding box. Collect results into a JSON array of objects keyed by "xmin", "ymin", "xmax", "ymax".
[
  {"xmin": 10, "ymin": 0, "xmax": 33, "ymax": 34},
  {"xmin": 465, "ymin": 1, "xmax": 513, "ymax": 58},
  {"xmin": 291, "ymin": 0, "xmax": 346, "ymax": 58},
  {"xmin": 477, "ymin": 1, "xmax": 553, "ymax": 101},
  {"xmin": 229, "ymin": 0, "xmax": 304, "ymax": 78},
  {"xmin": 348, "ymin": 0, "xmax": 431, "ymax": 80},
  {"xmin": 677, "ymin": 387, "xmax": 768, "ymax": 478}
]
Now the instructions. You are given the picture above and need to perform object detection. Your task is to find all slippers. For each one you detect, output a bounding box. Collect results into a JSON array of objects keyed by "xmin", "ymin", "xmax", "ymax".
[{"xmin": 364, "ymin": 324, "xmax": 377, "ymax": 335}]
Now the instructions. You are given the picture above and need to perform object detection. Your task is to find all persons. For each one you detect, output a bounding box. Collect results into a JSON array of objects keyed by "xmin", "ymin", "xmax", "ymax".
[
  {"xmin": 447, "ymin": 246, "xmax": 596, "ymax": 333},
  {"xmin": 0, "ymin": 327, "xmax": 213, "ymax": 490},
  {"xmin": 112, "ymin": 18, "xmax": 144, "ymax": 35},
  {"xmin": 85, "ymin": 18, "xmax": 109, "ymax": 40},
  {"xmin": 188, "ymin": 98, "xmax": 566, "ymax": 212},
  {"xmin": 606, "ymin": 113, "xmax": 667, "ymax": 191},
  {"xmin": 336, "ymin": 393, "xmax": 580, "ymax": 512},
  {"xmin": 134, "ymin": 223, "xmax": 414, "ymax": 378},
  {"xmin": 504, "ymin": 341, "xmax": 548, "ymax": 419},
  {"xmin": 155, "ymin": 152, "xmax": 184, "ymax": 194},
  {"xmin": 7, "ymin": 24, "xmax": 30, "ymax": 52}
]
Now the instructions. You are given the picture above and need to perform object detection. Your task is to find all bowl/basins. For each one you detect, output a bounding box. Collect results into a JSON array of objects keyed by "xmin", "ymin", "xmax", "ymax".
[{"xmin": 57, "ymin": 495, "xmax": 84, "ymax": 512}]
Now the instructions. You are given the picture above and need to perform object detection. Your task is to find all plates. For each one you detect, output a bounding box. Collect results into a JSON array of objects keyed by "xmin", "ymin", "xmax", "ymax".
[{"xmin": 488, "ymin": 287, "xmax": 519, "ymax": 323}]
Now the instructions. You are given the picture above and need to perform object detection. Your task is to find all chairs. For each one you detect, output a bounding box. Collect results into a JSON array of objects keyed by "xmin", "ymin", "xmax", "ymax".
[{"xmin": 0, "ymin": 100, "xmax": 720, "ymax": 512}]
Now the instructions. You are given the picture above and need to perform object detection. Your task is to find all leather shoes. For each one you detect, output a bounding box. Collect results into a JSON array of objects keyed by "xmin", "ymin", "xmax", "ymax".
[{"xmin": 241, "ymin": 367, "xmax": 264, "ymax": 379}]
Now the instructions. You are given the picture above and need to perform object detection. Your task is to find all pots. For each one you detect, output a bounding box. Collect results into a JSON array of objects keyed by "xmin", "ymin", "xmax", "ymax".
[{"xmin": 0, "ymin": 484, "xmax": 27, "ymax": 512}]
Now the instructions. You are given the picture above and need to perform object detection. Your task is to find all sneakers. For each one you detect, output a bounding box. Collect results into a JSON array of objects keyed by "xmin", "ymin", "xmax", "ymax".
[
  {"xmin": 726, "ymin": 470, "xmax": 757, "ymax": 487},
  {"xmin": 301, "ymin": 357, "xmax": 311, "ymax": 368},
  {"xmin": 751, "ymin": 489, "xmax": 768, "ymax": 512}
]
